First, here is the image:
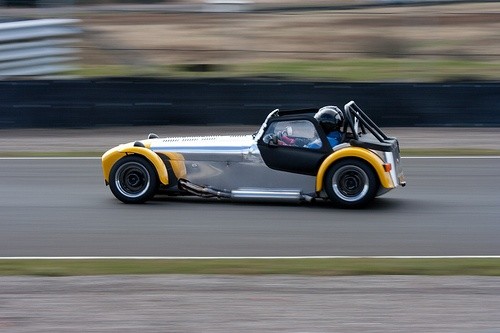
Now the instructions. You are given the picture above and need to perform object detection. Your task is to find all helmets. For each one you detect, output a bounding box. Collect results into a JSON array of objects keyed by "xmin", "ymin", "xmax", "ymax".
[{"xmin": 313, "ymin": 106, "xmax": 344, "ymax": 130}]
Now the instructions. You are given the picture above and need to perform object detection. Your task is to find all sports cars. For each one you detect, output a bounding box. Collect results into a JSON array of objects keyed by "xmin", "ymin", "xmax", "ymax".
[{"xmin": 103, "ymin": 100, "xmax": 404, "ymax": 210}]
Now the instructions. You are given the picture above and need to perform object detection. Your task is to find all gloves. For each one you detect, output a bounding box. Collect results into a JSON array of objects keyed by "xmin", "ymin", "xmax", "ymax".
[
  {"xmin": 281, "ymin": 134, "xmax": 294, "ymax": 144},
  {"xmin": 277, "ymin": 139, "xmax": 290, "ymax": 146}
]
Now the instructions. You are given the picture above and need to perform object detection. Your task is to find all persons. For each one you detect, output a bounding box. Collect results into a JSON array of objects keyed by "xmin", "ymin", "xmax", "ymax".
[{"xmin": 272, "ymin": 105, "xmax": 347, "ymax": 152}]
